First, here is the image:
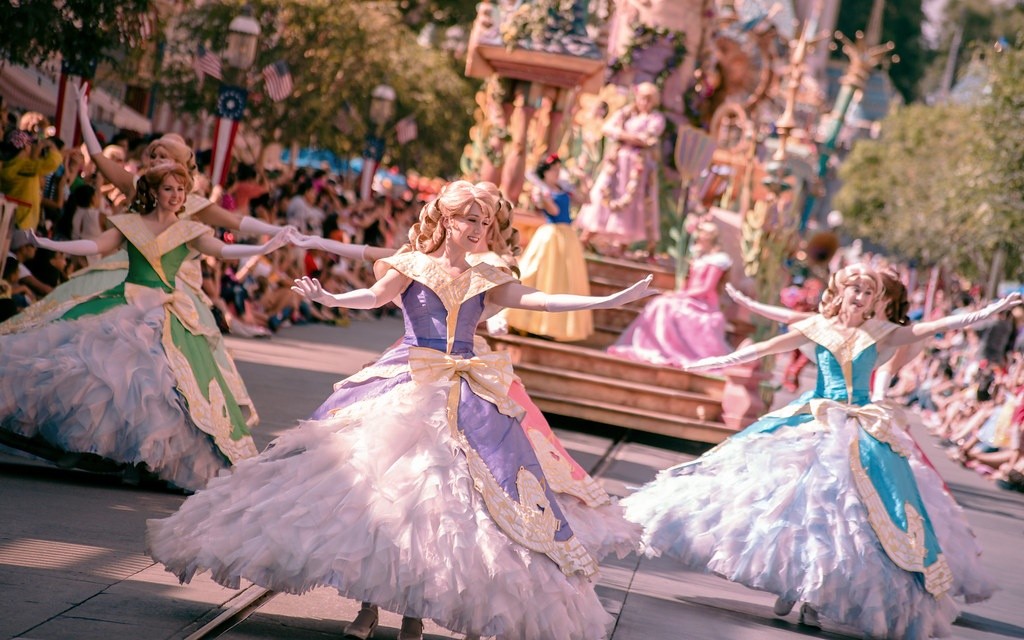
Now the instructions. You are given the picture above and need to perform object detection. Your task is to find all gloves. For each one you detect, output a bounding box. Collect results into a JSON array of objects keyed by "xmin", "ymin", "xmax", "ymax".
[
  {"xmin": 545, "ymin": 274, "xmax": 657, "ymax": 312},
  {"xmin": 240, "ymin": 216, "xmax": 296, "ymax": 241},
  {"xmin": 222, "ymin": 227, "xmax": 289, "ymax": 259},
  {"xmin": 24, "ymin": 228, "xmax": 97, "ymax": 256},
  {"xmin": 948, "ymin": 292, "xmax": 1022, "ymax": 330},
  {"xmin": 687, "ymin": 345, "xmax": 757, "ymax": 369},
  {"xmin": 290, "ymin": 232, "xmax": 368, "ymax": 261},
  {"xmin": 725, "ymin": 282, "xmax": 790, "ymax": 322},
  {"xmin": 291, "ymin": 276, "xmax": 376, "ymax": 309},
  {"xmin": 73, "ymin": 81, "xmax": 102, "ymax": 154}
]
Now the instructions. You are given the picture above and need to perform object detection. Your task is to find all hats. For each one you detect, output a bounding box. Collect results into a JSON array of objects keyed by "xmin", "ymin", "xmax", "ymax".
[{"xmin": 11, "ymin": 231, "xmax": 36, "ymax": 248}]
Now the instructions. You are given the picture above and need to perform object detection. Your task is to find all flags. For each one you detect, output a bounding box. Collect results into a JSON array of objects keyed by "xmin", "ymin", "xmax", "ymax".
[
  {"xmin": 193, "ymin": 44, "xmax": 224, "ymax": 80},
  {"xmin": 262, "ymin": 59, "xmax": 293, "ymax": 102},
  {"xmin": 394, "ymin": 117, "xmax": 418, "ymax": 145}
]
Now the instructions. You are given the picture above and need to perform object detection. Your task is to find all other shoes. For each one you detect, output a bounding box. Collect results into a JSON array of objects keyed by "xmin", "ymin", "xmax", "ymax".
[
  {"xmin": 122, "ymin": 471, "xmax": 144, "ymax": 486},
  {"xmin": 56, "ymin": 454, "xmax": 79, "ymax": 467},
  {"xmin": 512, "ymin": 327, "xmax": 528, "ymax": 336}
]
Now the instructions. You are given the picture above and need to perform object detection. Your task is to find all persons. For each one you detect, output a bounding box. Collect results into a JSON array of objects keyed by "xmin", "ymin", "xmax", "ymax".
[{"xmin": 0, "ymin": 83, "xmax": 1024, "ymax": 640}]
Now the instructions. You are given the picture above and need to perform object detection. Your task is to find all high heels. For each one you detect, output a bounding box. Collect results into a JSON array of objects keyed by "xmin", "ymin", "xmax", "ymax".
[
  {"xmin": 774, "ymin": 596, "xmax": 796, "ymax": 616},
  {"xmin": 397, "ymin": 617, "xmax": 424, "ymax": 640},
  {"xmin": 800, "ymin": 602, "xmax": 821, "ymax": 630},
  {"xmin": 343, "ymin": 608, "xmax": 378, "ymax": 640}
]
